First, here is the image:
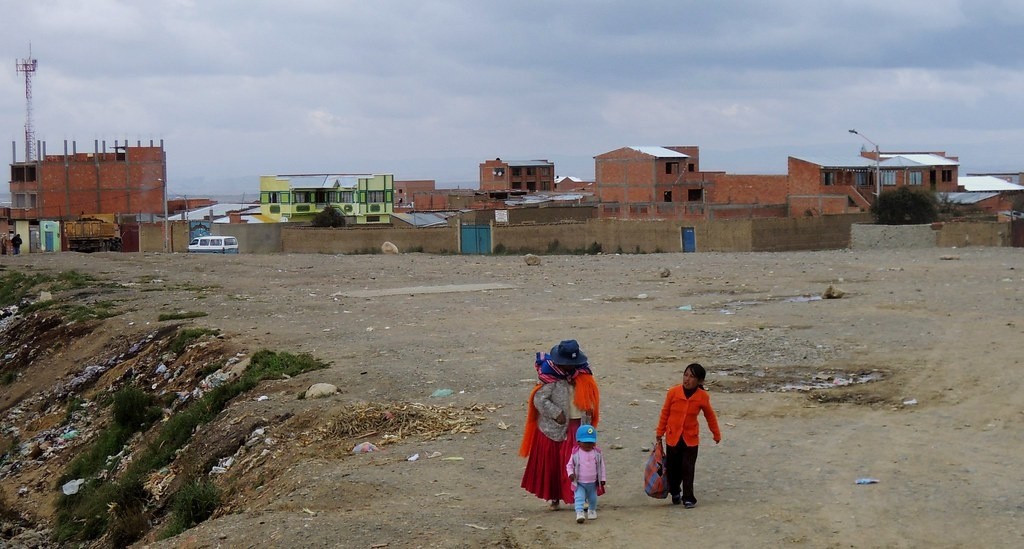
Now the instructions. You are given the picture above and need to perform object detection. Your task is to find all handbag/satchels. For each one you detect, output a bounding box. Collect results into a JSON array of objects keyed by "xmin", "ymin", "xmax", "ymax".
[{"xmin": 643, "ymin": 438, "xmax": 668, "ymax": 499}]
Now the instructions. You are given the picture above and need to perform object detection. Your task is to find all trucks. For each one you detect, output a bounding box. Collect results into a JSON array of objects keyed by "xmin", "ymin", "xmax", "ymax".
[{"xmin": 65, "ymin": 211, "xmax": 124, "ymax": 254}]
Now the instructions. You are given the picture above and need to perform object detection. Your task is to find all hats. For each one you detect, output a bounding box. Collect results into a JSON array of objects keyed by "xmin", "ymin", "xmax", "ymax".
[
  {"xmin": 550, "ymin": 339, "xmax": 587, "ymax": 366},
  {"xmin": 576, "ymin": 425, "xmax": 597, "ymax": 442}
]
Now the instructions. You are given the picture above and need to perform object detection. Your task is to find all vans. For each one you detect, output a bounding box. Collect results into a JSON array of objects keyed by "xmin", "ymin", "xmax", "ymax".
[{"xmin": 188, "ymin": 235, "xmax": 239, "ymax": 254}]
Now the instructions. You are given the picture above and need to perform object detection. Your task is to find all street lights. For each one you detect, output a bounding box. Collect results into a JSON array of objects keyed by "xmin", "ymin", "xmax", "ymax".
[
  {"xmin": 157, "ymin": 177, "xmax": 168, "ymax": 253},
  {"xmin": 849, "ymin": 129, "xmax": 880, "ymax": 204}
]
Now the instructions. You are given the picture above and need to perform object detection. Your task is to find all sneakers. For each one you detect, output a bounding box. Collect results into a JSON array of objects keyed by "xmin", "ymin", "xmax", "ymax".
[
  {"xmin": 588, "ymin": 509, "xmax": 598, "ymax": 520},
  {"xmin": 576, "ymin": 511, "xmax": 585, "ymax": 524}
]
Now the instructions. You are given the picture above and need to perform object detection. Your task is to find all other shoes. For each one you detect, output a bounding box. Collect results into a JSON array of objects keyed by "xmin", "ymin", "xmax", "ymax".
[
  {"xmin": 683, "ymin": 498, "xmax": 693, "ymax": 509},
  {"xmin": 549, "ymin": 502, "xmax": 559, "ymax": 511},
  {"xmin": 672, "ymin": 493, "xmax": 681, "ymax": 505}
]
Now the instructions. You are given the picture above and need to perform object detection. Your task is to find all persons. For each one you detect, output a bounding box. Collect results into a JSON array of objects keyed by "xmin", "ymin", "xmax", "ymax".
[
  {"xmin": 518, "ymin": 339, "xmax": 607, "ymax": 523},
  {"xmin": 1, "ymin": 233, "xmax": 8, "ymax": 254},
  {"xmin": 11, "ymin": 233, "xmax": 22, "ymax": 255},
  {"xmin": 655, "ymin": 363, "xmax": 721, "ymax": 507}
]
